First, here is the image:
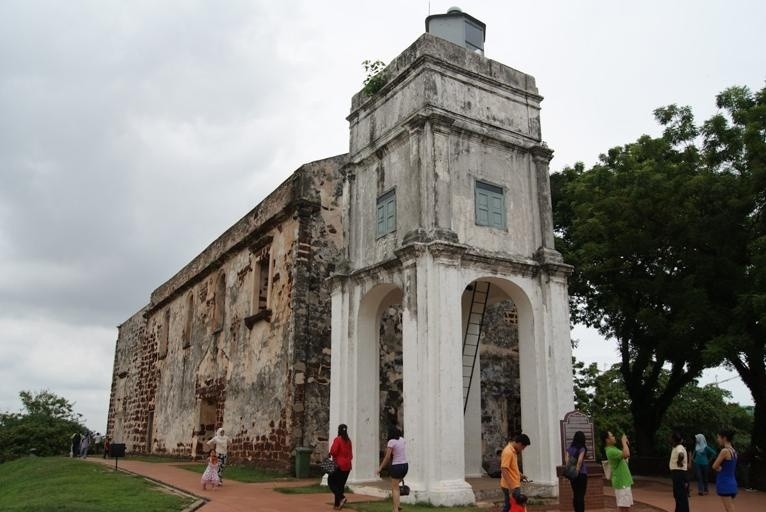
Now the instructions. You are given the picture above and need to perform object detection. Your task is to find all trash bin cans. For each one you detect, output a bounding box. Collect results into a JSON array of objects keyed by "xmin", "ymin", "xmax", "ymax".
[
  {"xmin": 294, "ymin": 447, "xmax": 313, "ymax": 478},
  {"xmin": 109, "ymin": 444, "xmax": 127, "ymax": 457}
]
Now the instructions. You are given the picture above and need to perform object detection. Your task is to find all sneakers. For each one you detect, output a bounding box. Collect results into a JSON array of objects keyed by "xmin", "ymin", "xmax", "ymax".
[{"xmin": 335, "ymin": 499, "xmax": 347, "ymax": 509}]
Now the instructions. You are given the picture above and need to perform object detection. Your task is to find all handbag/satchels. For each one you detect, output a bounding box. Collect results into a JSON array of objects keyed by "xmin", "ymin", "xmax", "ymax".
[
  {"xmin": 399, "ymin": 480, "xmax": 410, "ymax": 496},
  {"xmin": 320, "ymin": 453, "xmax": 336, "ymax": 474},
  {"xmin": 564, "ymin": 457, "xmax": 582, "ymax": 478},
  {"xmin": 601, "ymin": 460, "xmax": 610, "ymax": 480}
]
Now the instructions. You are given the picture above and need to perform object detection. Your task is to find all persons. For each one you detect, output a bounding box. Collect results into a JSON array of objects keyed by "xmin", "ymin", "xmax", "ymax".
[
  {"xmin": 710, "ymin": 426, "xmax": 739, "ymax": 512},
  {"xmin": 667, "ymin": 432, "xmax": 692, "ymax": 512},
  {"xmin": 68, "ymin": 429, "xmax": 101, "ymax": 458},
  {"xmin": 322, "ymin": 421, "xmax": 355, "ymax": 512},
  {"xmin": 498, "ymin": 434, "xmax": 530, "ymax": 512},
  {"xmin": 200, "ymin": 448, "xmax": 221, "ymax": 493},
  {"xmin": 599, "ymin": 430, "xmax": 635, "ymax": 512},
  {"xmin": 564, "ymin": 431, "xmax": 588, "ymax": 512},
  {"xmin": 205, "ymin": 427, "xmax": 232, "ymax": 483},
  {"xmin": 690, "ymin": 434, "xmax": 717, "ymax": 496},
  {"xmin": 506, "ymin": 489, "xmax": 527, "ymax": 512},
  {"xmin": 374, "ymin": 427, "xmax": 409, "ymax": 512},
  {"xmin": 674, "ymin": 433, "xmax": 693, "ymax": 498}
]
